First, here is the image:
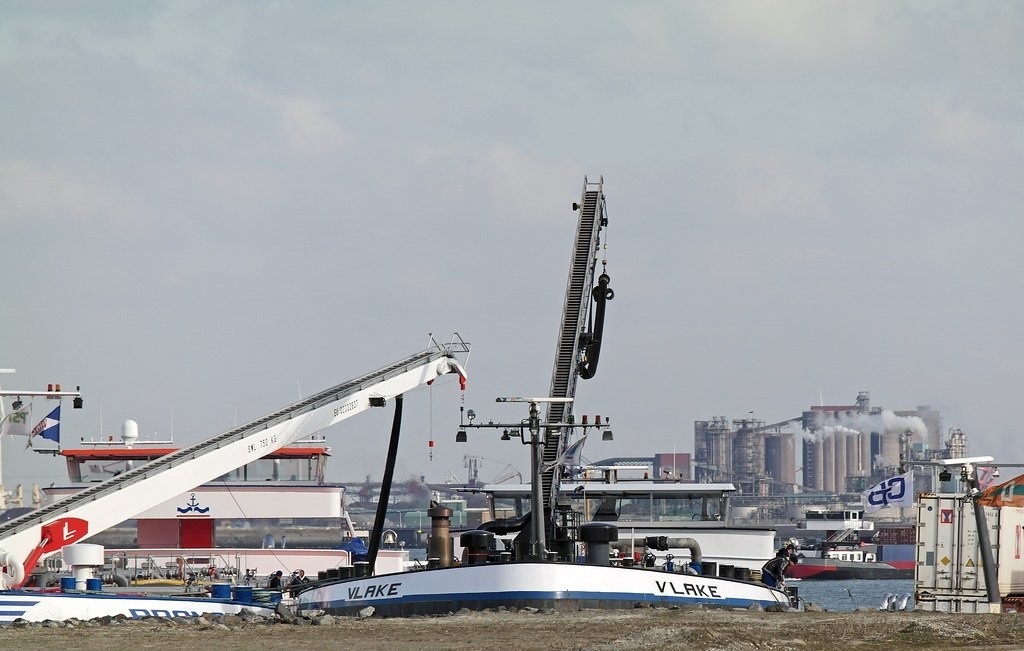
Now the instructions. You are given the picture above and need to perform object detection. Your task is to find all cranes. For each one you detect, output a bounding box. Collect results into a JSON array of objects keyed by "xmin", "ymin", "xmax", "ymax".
[
  {"xmin": 0, "ymin": 333, "xmax": 472, "ymax": 592},
  {"xmin": 295, "ymin": 172, "xmax": 800, "ymax": 618}
]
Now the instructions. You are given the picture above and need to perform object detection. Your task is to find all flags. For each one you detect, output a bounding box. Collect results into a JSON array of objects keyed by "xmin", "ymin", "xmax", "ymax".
[
  {"xmin": 978, "ymin": 474, "xmax": 1024, "ymax": 508},
  {"xmin": 542, "ymin": 435, "xmax": 587, "ymax": 472},
  {"xmin": 0, "ymin": 402, "xmax": 32, "ymax": 436},
  {"xmin": 32, "ymin": 404, "xmax": 59, "ymax": 443},
  {"xmin": 860, "ymin": 470, "xmax": 914, "ymax": 513}
]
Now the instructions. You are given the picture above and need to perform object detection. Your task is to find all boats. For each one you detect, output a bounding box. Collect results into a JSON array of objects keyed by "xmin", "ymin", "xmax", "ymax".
[
  {"xmin": 0, "ymin": 383, "xmax": 428, "ymax": 622},
  {"xmin": 783, "ymin": 510, "xmax": 915, "ymax": 579},
  {"xmin": 453, "ymin": 444, "xmax": 777, "ymax": 572}
]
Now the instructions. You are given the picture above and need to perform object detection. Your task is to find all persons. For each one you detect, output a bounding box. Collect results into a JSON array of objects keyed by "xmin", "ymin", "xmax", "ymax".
[
  {"xmin": 761, "ymin": 556, "xmax": 799, "ymax": 589},
  {"xmin": 269, "ymin": 570, "xmax": 282, "ymax": 588},
  {"xmin": 292, "ymin": 570, "xmax": 311, "ymax": 585},
  {"xmin": 776, "ymin": 544, "xmax": 795, "ymax": 559}
]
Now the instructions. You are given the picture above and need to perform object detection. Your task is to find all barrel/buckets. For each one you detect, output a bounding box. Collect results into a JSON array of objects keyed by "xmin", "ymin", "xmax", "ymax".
[
  {"xmin": 702, "ymin": 562, "xmax": 717, "ymax": 576},
  {"xmin": 689, "ymin": 562, "xmax": 700, "ymax": 573},
  {"xmin": 428, "ymin": 558, "xmax": 440, "ymax": 568},
  {"xmin": 210, "ymin": 583, "xmax": 231, "ymax": 599},
  {"xmin": 318, "ymin": 571, "xmax": 328, "ymax": 581},
  {"xmin": 86, "ymin": 578, "xmax": 103, "ymax": 593},
  {"xmin": 719, "ymin": 564, "xmax": 734, "ymax": 578},
  {"xmin": 353, "ymin": 562, "xmax": 370, "ymax": 577},
  {"xmin": 268, "ymin": 591, "xmax": 283, "ymax": 604},
  {"xmin": 61, "ymin": 577, "xmax": 76, "ymax": 589},
  {"xmin": 338, "ymin": 566, "xmax": 354, "ymax": 577},
  {"xmin": 234, "ymin": 586, "xmax": 252, "ymax": 602},
  {"xmin": 734, "ymin": 567, "xmax": 750, "ymax": 582},
  {"xmin": 327, "ymin": 568, "xmax": 339, "ymax": 577}
]
